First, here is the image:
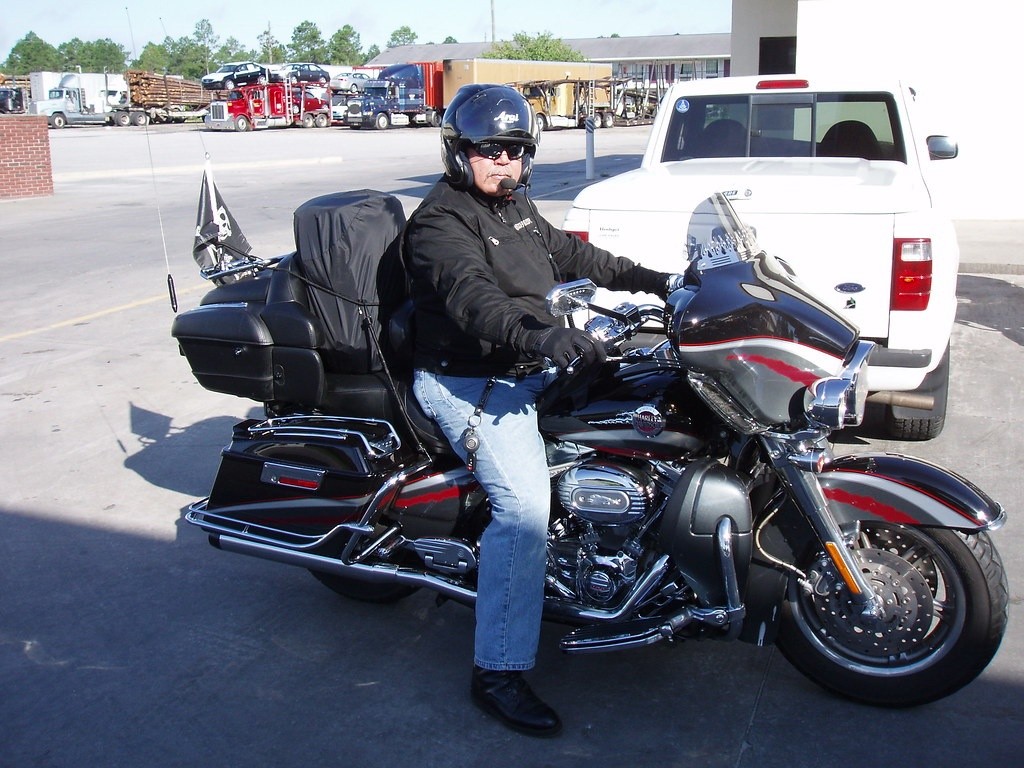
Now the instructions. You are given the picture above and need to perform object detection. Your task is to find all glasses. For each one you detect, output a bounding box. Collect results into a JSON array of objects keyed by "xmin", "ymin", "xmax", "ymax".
[{"xmin": 473, "ymin": 143, "xmax": 528, "ymax": 160}]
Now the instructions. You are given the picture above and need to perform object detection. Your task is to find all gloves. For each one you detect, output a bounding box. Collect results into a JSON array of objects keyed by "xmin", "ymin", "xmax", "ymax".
[
  {"xmin": 648, "ymin": 270, "xmax": 684, "ymax": 303},
  {"xmin": 518, "ymin": 317, "xmax": 613, "ymax": 381}
]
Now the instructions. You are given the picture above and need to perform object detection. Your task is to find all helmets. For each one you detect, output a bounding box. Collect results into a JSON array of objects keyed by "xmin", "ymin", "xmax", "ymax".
[{"xmin": 440, "ymin": 83, "xmax": 540, "ymax": 190}]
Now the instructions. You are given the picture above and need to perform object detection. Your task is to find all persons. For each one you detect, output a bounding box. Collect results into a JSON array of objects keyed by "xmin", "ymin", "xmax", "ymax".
[{"xmin": 404, "ymin": 83, "xmax": 683, "ymax": 740}]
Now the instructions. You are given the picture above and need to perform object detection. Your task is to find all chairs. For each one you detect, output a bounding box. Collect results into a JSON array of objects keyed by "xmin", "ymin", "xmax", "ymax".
[
  {"xmin": 818, "ymin": 119, "xmax": 886, "ymax": 161},
  {"xmin": 688, "ymin": 118, "xmax": 760, "ymax": 158}
]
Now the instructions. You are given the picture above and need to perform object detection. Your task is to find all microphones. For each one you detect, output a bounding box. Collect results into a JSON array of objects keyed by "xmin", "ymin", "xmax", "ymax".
[{"xmin": 500, "ymin": 179, "xmax": 526, "ymax": 190}]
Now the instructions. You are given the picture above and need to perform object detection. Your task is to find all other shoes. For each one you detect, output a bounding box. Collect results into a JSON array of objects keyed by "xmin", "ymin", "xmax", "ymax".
[{"xmin": 471, "ymin": 663, "xmax": 562, "ymax": 737}]
